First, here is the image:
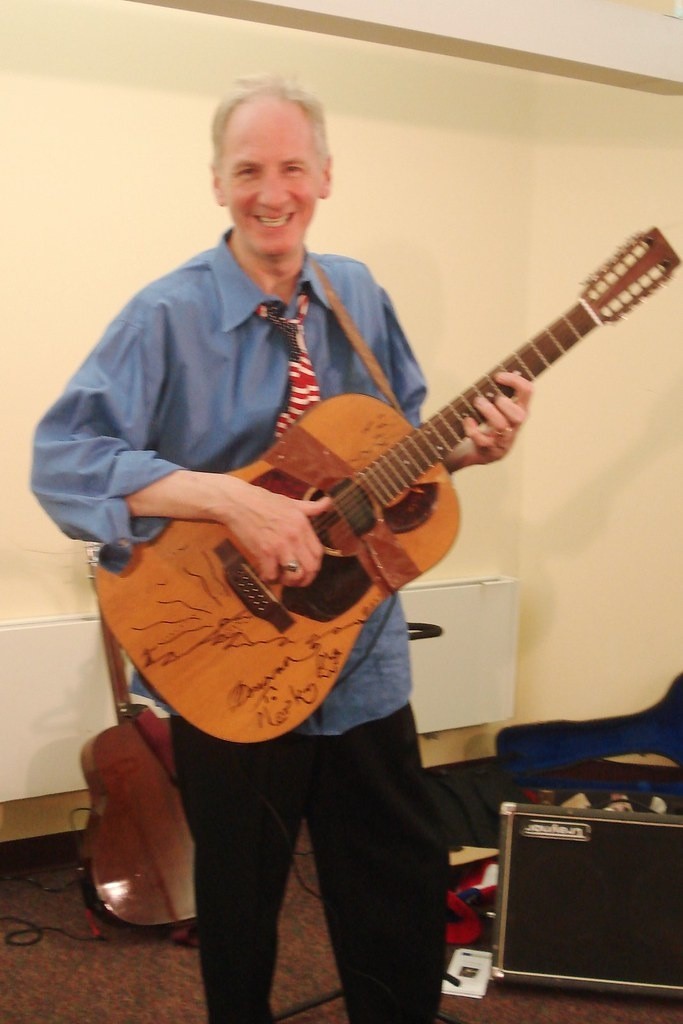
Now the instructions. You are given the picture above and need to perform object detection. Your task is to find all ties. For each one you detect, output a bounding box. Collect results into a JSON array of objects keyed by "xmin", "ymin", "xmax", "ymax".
[{"xmin": 253, "ymin": 280, "xmax": 322, "ymax": 441}]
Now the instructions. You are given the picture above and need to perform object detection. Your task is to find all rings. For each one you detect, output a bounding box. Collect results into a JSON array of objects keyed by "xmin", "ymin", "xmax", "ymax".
[
  {"xmin": 496, "ymin": 427, "xmax": 512, "ymax": 437},
  {"xmin": 283, "ymin": 560, "xmax": 299, "ymax": 573}
]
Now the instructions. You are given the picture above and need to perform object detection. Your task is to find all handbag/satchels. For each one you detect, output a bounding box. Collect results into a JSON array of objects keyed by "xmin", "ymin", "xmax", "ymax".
[{"xmin": 427, "ymin": 766, "xmax": 536, "ymax": 846}]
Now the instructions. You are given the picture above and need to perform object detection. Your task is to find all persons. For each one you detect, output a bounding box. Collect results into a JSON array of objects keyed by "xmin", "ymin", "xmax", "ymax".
[{"xmin": 30, "ymin": 82, "xmax": 534, "ymax": 1024}]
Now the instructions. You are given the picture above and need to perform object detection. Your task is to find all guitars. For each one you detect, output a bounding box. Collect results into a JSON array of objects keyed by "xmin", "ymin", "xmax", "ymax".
[
  {"xmin": 78, "ymin": 542, "xmax": 199, "ymax": 929},
  {"xmin": 90, "ymin": 224, "xmax": 682, "ymax": 746}
]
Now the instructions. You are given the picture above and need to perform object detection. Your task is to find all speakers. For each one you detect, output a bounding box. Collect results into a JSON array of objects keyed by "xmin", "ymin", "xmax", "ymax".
[{"xmin": 492, "ymin": 801, "xmax": 683, "ymax": 1002}]
